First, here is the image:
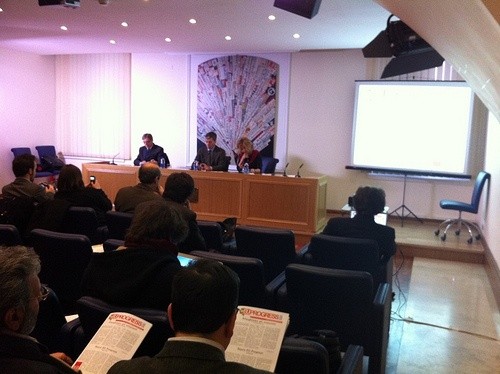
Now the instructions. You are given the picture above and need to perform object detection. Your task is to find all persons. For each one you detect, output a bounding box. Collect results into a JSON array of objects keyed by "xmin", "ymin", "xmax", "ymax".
[
  {"xmin": 106, "ymin": 260, "xmax": 275, "ymax": 374},
  {"xmin": 235, "ymin": 137, "xmax": 262, "ymax": 174},
  {"xmin": 115, "ymin": 163, "xmax": 165, "ymax": 213},
  {"xmin": 0, "ymin": 246, "xmax": 82, "ymax": 374},
  {"xmin": 191, "ymin": 132, "xmax": 229, "ymax": 172},
  {"xmin": 134, "ymin": 133, "xmax": 170, "ymax": 168},
  {"xmin": 2, "ymin": 154, "xmax": 54, "ymax": 202},
  {"xmin": 134, "ymin": 173, "xmax": 208, "ymax": 252},
  {"xmin": 79, "ymin": 205, "xmax": 189, "ymax": 312},
  {"xmin": 54, "ymin": 165, "xmax": 112, "ymax": 212},
  {"xmin": 321, "ymin": 187, "xmax": 395, "ymax": 263}
]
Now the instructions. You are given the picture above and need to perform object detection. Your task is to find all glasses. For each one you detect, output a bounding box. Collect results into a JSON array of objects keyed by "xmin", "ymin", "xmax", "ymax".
[{"xmin": 28, "ymin": 286, "xmax": 50, "ymax": 301}]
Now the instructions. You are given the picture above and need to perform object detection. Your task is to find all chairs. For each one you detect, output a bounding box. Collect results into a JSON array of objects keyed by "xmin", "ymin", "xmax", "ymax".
[
  {"xmin": 0, "ymin": 206, "xmax": 395, "ymax": 374},
  {"xmin": 434, "ymin": 170, "xmax": 490, "ymax": 244}
]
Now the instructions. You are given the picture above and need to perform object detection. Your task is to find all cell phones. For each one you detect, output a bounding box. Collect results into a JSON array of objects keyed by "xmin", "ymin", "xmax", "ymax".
[{"xmin": 90, "ymin": 176, "xmax": 95, "ymax": 184}]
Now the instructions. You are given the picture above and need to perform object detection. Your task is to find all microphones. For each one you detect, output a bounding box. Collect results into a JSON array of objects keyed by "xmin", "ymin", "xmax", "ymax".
[
  {"xmin": 294, "ymin": 164, "xmax": 304, "ymax": 178},
  {"xmin": 283, "ymin": 163, "xmax": 289, "ymax": 177},
  {"xmin": 110, "ymin": 153, "xmax": 120, "ymax": 166}
]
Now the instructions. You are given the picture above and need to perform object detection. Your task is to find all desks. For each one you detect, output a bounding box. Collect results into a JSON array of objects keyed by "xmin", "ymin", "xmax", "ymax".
[{"xmin": 83, "ymin": 161, "xmax": 328, "ymax": 234}]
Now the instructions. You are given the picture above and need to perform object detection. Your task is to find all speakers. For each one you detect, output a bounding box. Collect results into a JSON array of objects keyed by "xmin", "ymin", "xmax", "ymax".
[{"xmin": 273, "ymin": 0, "xmax": 323, "ymax": 19}]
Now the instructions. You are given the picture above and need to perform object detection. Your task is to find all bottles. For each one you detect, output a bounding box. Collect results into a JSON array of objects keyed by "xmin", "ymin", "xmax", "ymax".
[
  {"xmin": 160, "ymin": 158, "xmax": 165, "ymax": 169},
  {"xmin": 193, "ymin": 160, "xmax": 198, "ymax": 170},
  {"xmin": 242, "ymin": 163, "xmax": 249, "ymax": 174}
]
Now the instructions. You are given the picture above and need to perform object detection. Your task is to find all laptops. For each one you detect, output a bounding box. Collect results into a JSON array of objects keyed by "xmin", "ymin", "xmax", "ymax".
[
  {"xmin": 176, "ymin": 253, "xmax": 223, "ymax": 268},
  {"xmin": 351, "ymin": 211, "xmax": 387, "ymax": 227}
]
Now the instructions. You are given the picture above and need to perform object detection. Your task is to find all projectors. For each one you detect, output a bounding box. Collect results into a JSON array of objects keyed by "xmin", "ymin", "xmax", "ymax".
[{"xmin": 38, "ymin": 0, "xmax": 80, "ymax": 10}]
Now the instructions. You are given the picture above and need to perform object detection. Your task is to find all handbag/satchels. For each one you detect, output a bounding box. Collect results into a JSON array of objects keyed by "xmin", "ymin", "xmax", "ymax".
[
  {"xmin": 41, "ymin": 153, "xmax": 65, "ymax": 174},
  {"xmin": 216, "ymin": 217, "xmax": 238, "ymax": 242}
]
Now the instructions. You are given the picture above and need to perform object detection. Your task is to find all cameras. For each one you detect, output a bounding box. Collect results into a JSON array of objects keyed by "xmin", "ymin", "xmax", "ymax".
[{"xmin": 40, "ymin": 182, "xmax": 56, "ymax": 190}]
